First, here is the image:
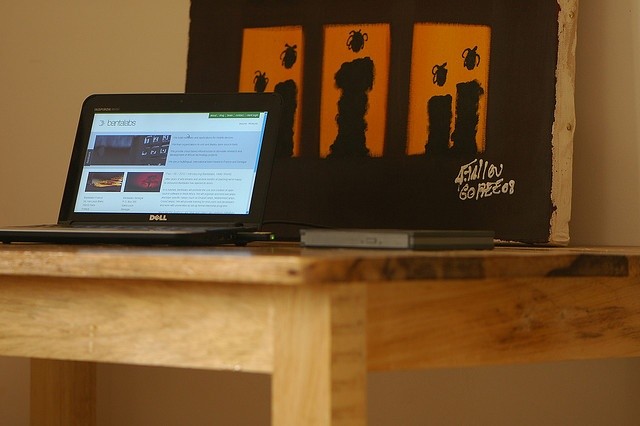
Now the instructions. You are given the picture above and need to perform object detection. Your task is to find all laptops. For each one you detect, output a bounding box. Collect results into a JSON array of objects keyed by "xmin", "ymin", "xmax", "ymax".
[{"xmin": 1, "ymin": 93, "xmax": 285, "ymax": 247}]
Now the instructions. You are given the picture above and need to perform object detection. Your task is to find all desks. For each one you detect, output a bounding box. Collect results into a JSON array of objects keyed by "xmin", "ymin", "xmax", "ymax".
[{"xmin": 2, "ymin": 245, "xmax": 638, "ymax": 425}]
